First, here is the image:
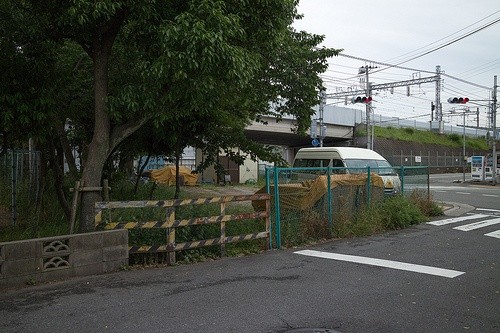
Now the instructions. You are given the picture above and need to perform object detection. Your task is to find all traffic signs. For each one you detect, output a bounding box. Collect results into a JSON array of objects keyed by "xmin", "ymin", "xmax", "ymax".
[{"xmin": 311, "ymin": 139, "xmax": 319, "ymax": 147}]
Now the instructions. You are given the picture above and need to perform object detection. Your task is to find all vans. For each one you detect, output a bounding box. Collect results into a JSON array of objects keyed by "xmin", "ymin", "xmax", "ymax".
[{"xmin": 291, "ymin": 147, "xmax": 402, "ymax": 197}]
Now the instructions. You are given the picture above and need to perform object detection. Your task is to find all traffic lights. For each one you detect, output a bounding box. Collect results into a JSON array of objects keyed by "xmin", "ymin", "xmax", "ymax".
[
  {"xmin": 447, "ymin": 97, "xmax": 469, "ymax": 104},
  {"xmin": 351, "ymin": 96, "xmax": 372, "ymax": 104}
]
